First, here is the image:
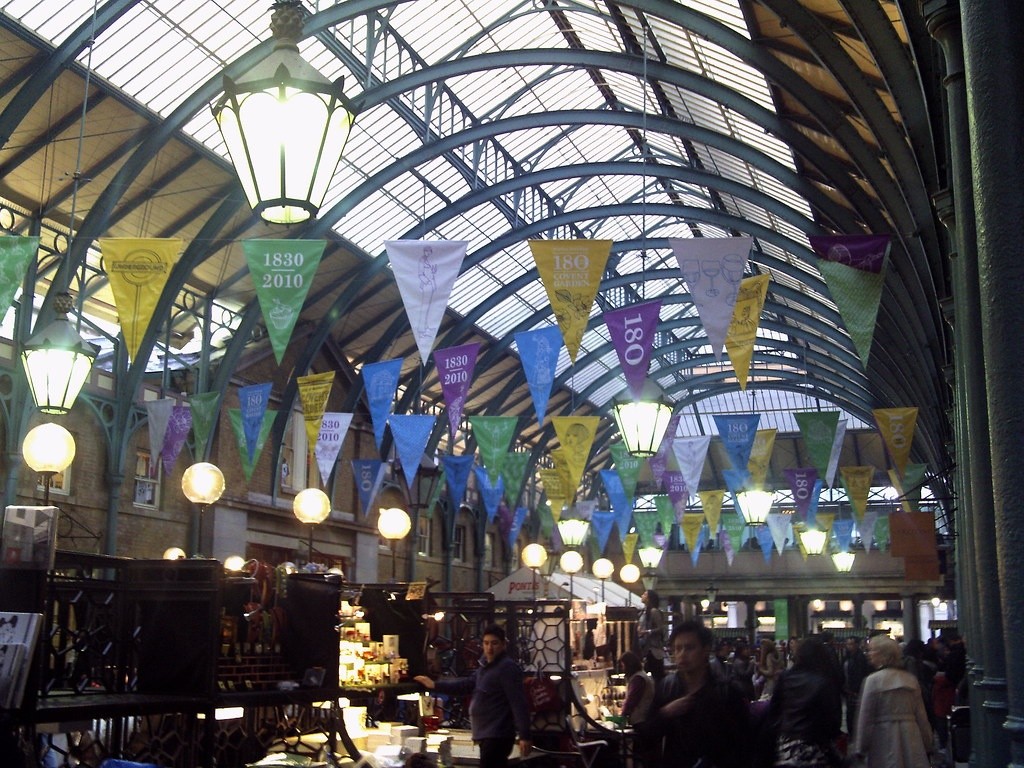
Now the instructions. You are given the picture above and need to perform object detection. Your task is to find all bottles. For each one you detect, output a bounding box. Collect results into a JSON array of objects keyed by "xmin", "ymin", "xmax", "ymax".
[{"xmin": 601, "ymin": 692, "xmax": 626, "ymax": 716}]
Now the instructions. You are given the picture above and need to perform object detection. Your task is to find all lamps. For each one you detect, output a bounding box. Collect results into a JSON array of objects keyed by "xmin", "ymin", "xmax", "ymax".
[
  {"xmin": 560, "ymin": 551, "xmax": 585, "ymax": 574},
  {"xmin": 619, "ymin": 564, "xmax": 641, "ymax": 584},
  {"xmin": 208, "ymin": 0, "xmax": 365, "ymax": 226},
  {"xmin": 610, "ymin": 25, "xmax": 674, "ymax": 460},
  {"xmin": 181, "ymin": 462, "xmax": 225, "ymax": 507},
  {"xmin": 557, "ymin": 371, "xmax": 590, "ymax": 547},
  {"xmin": 636, "ymin": 544, "xmax": 664, "ymax": 593},
  {"xmin": 292, "ymin": 488, "xmax": 330, "ymax": 526},
  {"xmin": 21, "ymin": 423, "xmax": 76, "ymax": 478},
  {"xmin": 18, "ymin": 0, "xmax": 101, "ymax": 415},
  {"xmin": 592, "ymin": 558, "xmax": 614, "ymax": 579},
  {"xmin": 733, "ymin": 350, "xmax": 775, "ymax": 526},
  {"xmin": 378, "ymin": 507, "xmax": 411, "ymax": 541},
  {"xmin": 798, "ymin": 528, "xmax": 856, "ymax": 574},
  {"xmin": 521, "ymin": 542, "xmax": 547, "ymax": 570},
  {"xmin": 704, "ymin": 583, "xmax": 718, "ymax": 604}
]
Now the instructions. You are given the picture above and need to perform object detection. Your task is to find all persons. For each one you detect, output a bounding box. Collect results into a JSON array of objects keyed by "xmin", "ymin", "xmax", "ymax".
[
  {"xmin": 413, "ymin": 625, "xmax": 533, "ymax": 768},
  {"xmin": 618, "ymin": 588, "xmax": 973, "ymax": 768}
]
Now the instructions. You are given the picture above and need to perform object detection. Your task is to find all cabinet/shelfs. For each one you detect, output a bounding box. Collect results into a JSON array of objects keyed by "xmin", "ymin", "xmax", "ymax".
[{"xmin": 1, "ymin": 541, "xmax": 641, "ymax": 768}]
